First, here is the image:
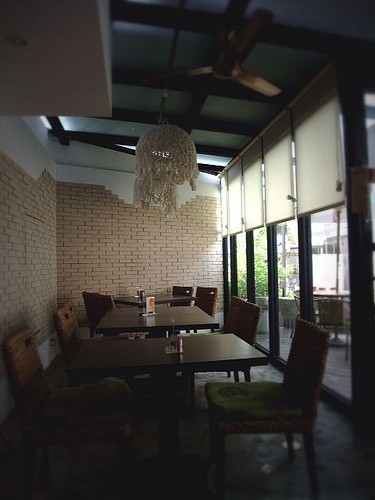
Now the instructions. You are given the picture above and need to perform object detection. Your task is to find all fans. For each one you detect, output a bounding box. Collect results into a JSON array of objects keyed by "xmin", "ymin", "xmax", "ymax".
[{"xmin": 165, "ymin": 9, "xmax": 282, "ymax": 97}]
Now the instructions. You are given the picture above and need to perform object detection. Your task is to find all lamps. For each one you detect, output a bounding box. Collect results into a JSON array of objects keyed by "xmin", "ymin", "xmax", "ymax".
[
  {"xmin": 134, "ymin": 166, "xmax": 178, "ymax": 218},
  {"xmin": 135, "ymin": 96, "xmax": 198, "ymax": 190}
]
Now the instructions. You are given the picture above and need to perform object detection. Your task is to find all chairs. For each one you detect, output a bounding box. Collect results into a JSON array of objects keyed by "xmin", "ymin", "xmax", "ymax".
[
  {"xmin": 54, "ymin": 303, "xmax": 94, "ymax": 386},
  {"xmin": 170, "ymin": 286, "xmax": 192, "ymax": 306},
  {"xmin": 222, "ymin": 296, "xmax": 242, "ymax": 336},
  {"xmin": 95, "ymin": 294, "xmax": 114, "ymax": 322},
  {"xmin": 195, "ymin": 287, "xmax": 216, "ymax": 317},
  {"xmin": 2, "ymin": 328, "xmax": 135, "ymax": 499},
  {"xmin": 230, "ymin": 300, "xmax": 261, "ymax": 383},
  {"xmin": 205, "ymin": 319, "xmax": 329, "ymax": 499},
  {"xmin": 290, "ymin": 290, "xmax": 302, "ymax": 337},
  {"xmin": 316, "ymin": 300, "xmax": 345, "ymax": 347},
  {"xmin": 82, "ymin": 292, "xmax": 99, "ymax": 338}
]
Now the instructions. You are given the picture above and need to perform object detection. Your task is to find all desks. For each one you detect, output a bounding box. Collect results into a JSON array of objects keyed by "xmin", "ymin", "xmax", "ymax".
[
  {"xmin": 314, "ymin": 289, "xmax": 351, "ymax": 297},
  {"xmin": 113, "ymin": 293, "xmax": 198, "ymax": 306},
  {"xmin": 70, "ymin": 336, "xmax": 269, "ymax": 480},
  {"xmin": 96, "ymin": 305, "xmax": 219, "ymax": 337}
]
